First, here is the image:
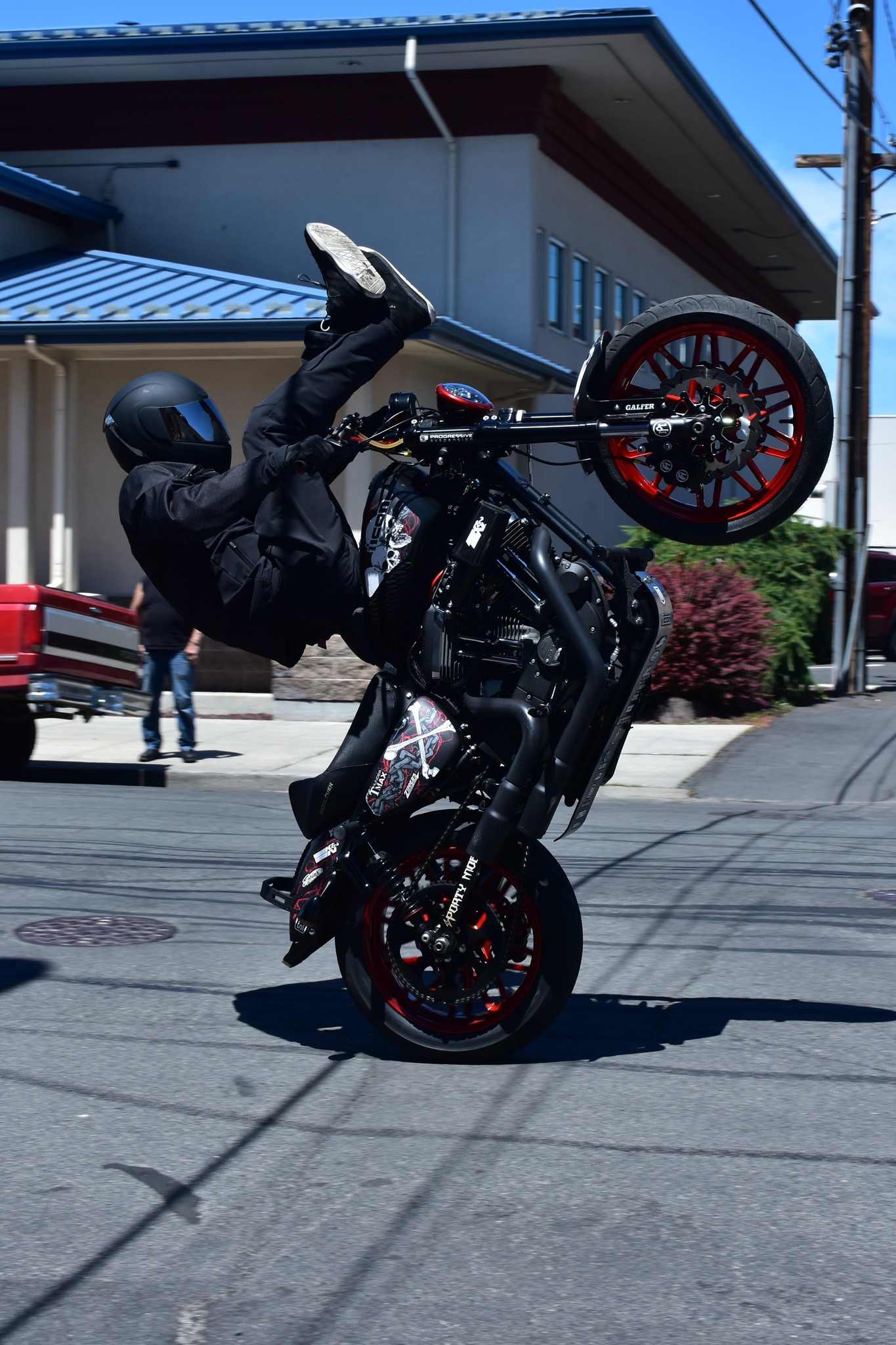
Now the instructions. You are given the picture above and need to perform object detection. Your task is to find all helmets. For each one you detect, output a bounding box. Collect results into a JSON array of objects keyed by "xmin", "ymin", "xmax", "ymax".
[{"xmin": 103, "ymin": 373, "xmax": 232, "ymax": 469}]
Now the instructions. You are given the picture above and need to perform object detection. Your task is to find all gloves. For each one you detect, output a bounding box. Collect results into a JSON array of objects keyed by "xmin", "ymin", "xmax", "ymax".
[{"xmin": 288, "ymin": 435, "xmax": 333, "ymax": 465}]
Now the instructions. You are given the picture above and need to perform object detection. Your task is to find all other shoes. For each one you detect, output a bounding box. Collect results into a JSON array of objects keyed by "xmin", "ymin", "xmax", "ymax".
[
  {"xmin": 141, "ymin": 749, "xmax": 160, "ymax": 762},
  {"xmin": 182, "ymin": 749, "xmax": 196, "ymax": 763}
]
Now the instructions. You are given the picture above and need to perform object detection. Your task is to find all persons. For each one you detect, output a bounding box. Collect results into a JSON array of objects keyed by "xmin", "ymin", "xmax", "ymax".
[
  {"xmin": 128, "ymin": 574, "xmax": 204, "ymax": 762},
  {"xmin": 100, "ymin": 222, "xmax": 438, "ymax": 667}
]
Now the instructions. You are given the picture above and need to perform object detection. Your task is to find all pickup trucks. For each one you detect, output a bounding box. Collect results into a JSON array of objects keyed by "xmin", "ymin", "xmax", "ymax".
[{"xmin": 0, "ymin": 580, "xmax": 153, "ymax": 779}]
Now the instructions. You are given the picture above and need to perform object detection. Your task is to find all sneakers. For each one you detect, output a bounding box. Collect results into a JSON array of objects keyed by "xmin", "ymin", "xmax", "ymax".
[
  {"xmin": 304, "ymin": 222, "xmax": 387, "ymax": 323},
  {"xmin": 358, "ymin": 245, "xmax": 439, "ymax": 338}
]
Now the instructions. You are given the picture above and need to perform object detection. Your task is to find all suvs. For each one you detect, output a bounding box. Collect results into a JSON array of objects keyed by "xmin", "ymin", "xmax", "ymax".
[{"xmin": 811, "ymin": 549, "xmax": 896, "ymax": 668}]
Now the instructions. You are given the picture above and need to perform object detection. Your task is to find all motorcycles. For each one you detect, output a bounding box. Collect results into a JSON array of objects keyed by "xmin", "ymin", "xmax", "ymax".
[{"xmin": 256, "ymin": 287, "xmax": 839, "ymax": 1066}]
